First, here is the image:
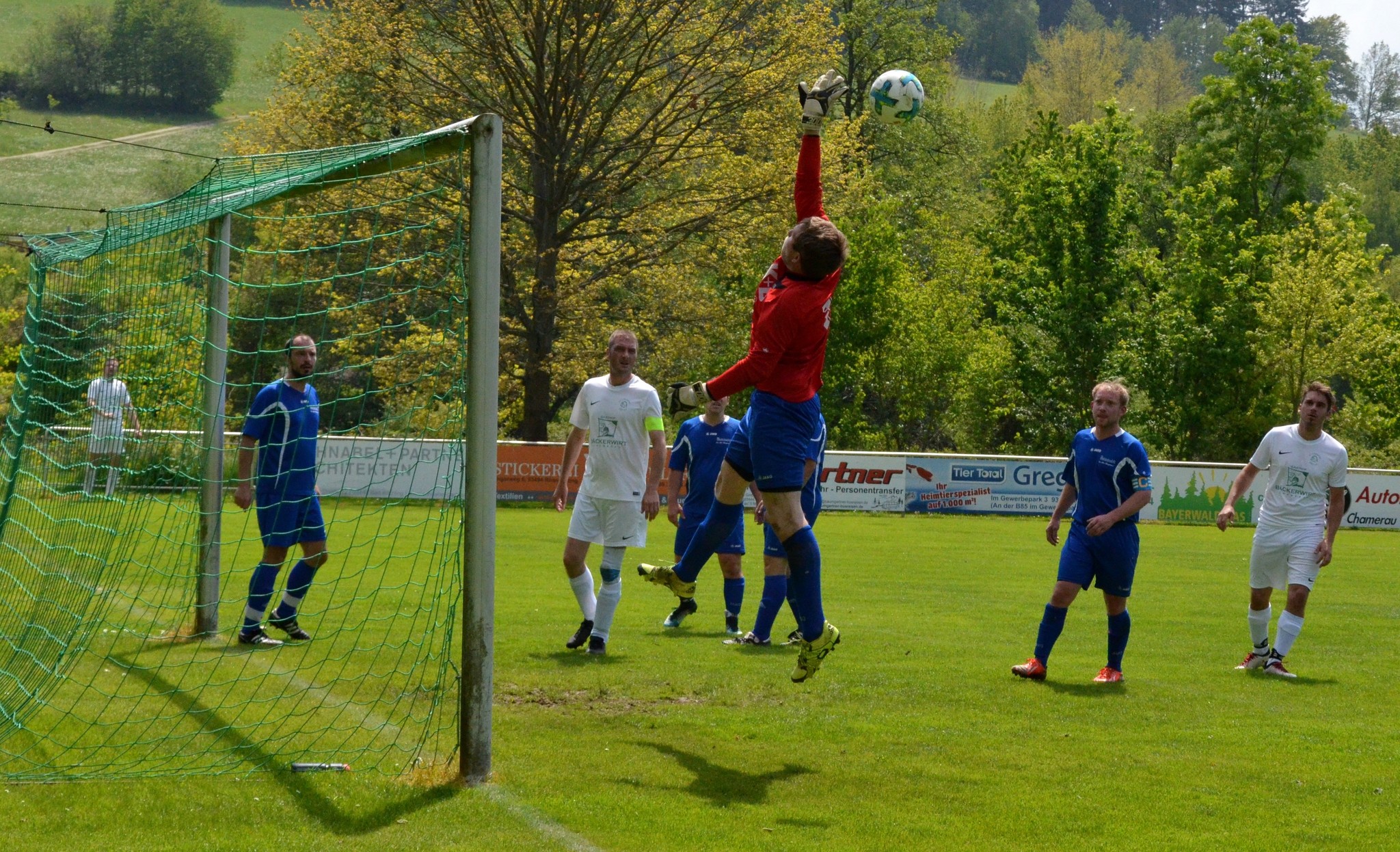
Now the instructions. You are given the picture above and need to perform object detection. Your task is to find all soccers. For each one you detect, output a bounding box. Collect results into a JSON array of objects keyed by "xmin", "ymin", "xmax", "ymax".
[{"xmin": 869, "ymin": 70, "xmax": 924, "ymax": 125}]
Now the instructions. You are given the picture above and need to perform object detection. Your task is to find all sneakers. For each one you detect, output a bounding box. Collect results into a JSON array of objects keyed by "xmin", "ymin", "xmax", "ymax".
[
  {"xmin": 238, "ymin": 630, "xmax": 283, "ymax": 647},
  {"xmin": 788, "ymin": 629, "xmax": 803, "ymax": 645},
  {"xmin": 663, "ymin": 597, "xmax": 697, "ymax": 627},
  {"xmin": 566, "ymin": 617, "xmax": 594, "ymax": 649},
  {"xmin": 268, "ymin": 607, "xmax": 311, "ymax": 640},
  {"xmin": 1092, "ymin": 666, "xmax": 1125, "ymax": 684},
  {"xmin": 1263, "ymin": 659, "xmax": 1296, "ymax": 680},
  {"xmin": 790, "ymin": 619, "xmax": 842, "ymax": 684},
  {"xmin": 1011, "ymin": 658, "xmax": 1047, "ymax": 682},
  {"xmin": 586, "ymin": 636, "xmax": 607, "ymax": 654},
  {"xmin": 723, "ymin": 610, "xmax": 743, "ymax": 637},
  {"xmin": 722, "ymin": 631, "xmax": 771, "ymax": 647},
  {"xmin": 637, "ymin": 559, "xmax": 697, "ymax": 599},
  {"xmin": 1235, "ymin": 649, "xmax": 1271, "ymax": 672}
]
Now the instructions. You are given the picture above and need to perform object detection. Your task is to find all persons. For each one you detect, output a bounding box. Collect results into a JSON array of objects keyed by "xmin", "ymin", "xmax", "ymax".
[
  {"xmin": 723, "ymin": 413, "xmax": 825, "ymax": 654},
  {"xmin": 1218, "ymin": 381, "xmax": 1347, "ymax": 677},
  {"xmin": 1012, "ymin": 383, "xmax": 1152, "ymax": 683},
  {"xmin": 235, "ymin": 336, "xmax": 328, "ymax": 647},
  {"xmin": 664, "ymin": 399, "xmax": 744, "ymax": 637},
  {"xmin": 636, "ymin": 68, "xmax": 887, "ymax": 682},
  {"xmin": 79, "ymin": 359, "xmax": 142, "ymax": 501},
  {"xmin": 554, "ymin": 329, "xmax": 665, "ymax": 654}
]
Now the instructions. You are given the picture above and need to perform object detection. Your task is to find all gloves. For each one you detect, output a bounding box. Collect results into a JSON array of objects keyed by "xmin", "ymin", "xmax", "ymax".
[
  {"xmin": 797, "ymin": 70, "xmax": 850, "ymax": 133},
  {"xmin": 665, "ymin": 379, "xmax": 712, "ymax": 418}
]
